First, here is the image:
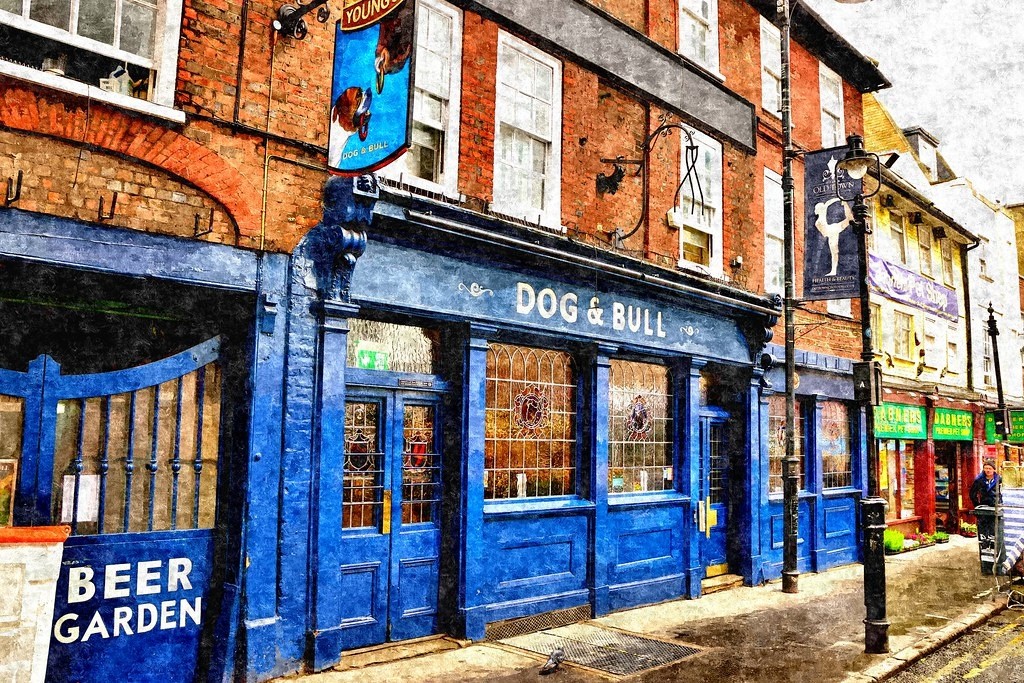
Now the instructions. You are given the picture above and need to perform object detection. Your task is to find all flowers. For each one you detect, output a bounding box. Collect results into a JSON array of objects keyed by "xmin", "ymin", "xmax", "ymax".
[{"xmin": 904, "ymin": 533, "xmax": 927, "ymax": 544}]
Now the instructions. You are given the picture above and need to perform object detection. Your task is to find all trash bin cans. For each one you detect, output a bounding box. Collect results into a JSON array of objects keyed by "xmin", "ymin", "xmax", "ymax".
[
  {"xmin": 0, "ymin": 523, "xmax": 74, "ymax": 683},
  {"xmin": 969, "ymin": 505, "xmax": 1006, "ymax": 575}
]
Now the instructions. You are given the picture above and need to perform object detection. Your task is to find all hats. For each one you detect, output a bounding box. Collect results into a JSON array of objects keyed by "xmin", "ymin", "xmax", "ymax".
[{"xmin": 983, "ymin": 461, "xmax": 995, "ymax": 470}]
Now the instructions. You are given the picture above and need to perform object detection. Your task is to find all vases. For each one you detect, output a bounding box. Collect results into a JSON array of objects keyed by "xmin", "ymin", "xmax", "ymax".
[{"xmin": 919, "ymin": 543, "xmax": 927, "ymax": 548}]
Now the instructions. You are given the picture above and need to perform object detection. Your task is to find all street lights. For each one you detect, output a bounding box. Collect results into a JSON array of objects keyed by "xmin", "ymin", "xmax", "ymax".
[{"xmin": 834, "ymin": 136, "xmax": 892, "ymax": 657}]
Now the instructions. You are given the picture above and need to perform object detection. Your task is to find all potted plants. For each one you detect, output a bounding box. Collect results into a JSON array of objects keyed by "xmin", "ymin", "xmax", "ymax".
[
  {"xmin": 924, "ymin": 531, "xmax": 951, "ymax": 546},
  {"xmin": 884, "ymin": 529, "xmax": 920, "ymax": 555}
]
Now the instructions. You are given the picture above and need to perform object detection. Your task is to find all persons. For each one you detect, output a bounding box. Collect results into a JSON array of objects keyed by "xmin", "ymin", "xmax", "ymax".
[{"xmin": 969, "ymin": 460, "xmax": 1002, "ymax": 507}]
